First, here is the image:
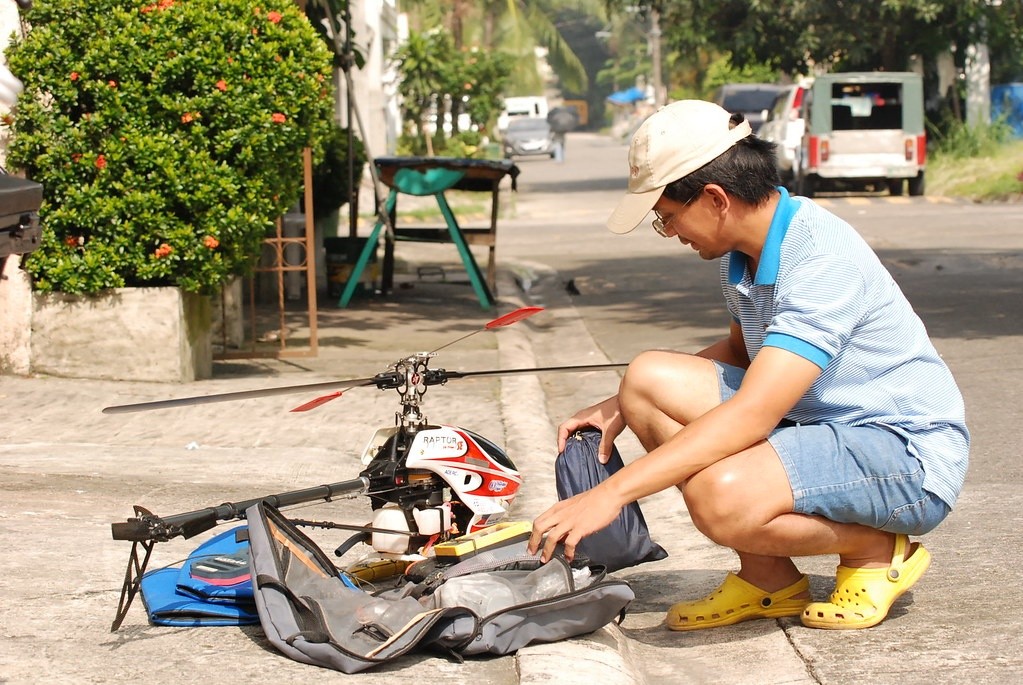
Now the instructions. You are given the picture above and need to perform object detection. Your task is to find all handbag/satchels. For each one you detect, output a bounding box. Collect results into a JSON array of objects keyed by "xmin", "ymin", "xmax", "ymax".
[{"xmin": 240, "ymin": 499, "xmax": 636, "ymax": 675}]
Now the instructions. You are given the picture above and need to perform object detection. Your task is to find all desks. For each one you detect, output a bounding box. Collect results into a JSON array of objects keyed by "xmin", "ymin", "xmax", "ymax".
[{"xmin": 370, "ymin": 152, "xmax": 521, "ymax": 297}]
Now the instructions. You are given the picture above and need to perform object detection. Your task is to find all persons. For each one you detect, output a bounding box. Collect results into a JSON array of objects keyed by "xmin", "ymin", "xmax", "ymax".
[
  {"xmin": 527, "ymin": 98, "xmax": 972, "ymax": 631},
  {"xmin": 547, "ymin": 97, "xmax": 569, "ymax": 163}
]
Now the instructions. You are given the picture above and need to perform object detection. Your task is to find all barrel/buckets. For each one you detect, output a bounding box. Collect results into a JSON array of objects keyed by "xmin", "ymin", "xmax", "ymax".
[{"xmin": 321, "ymin": 233, "xmax": 382, "ymax": 298}]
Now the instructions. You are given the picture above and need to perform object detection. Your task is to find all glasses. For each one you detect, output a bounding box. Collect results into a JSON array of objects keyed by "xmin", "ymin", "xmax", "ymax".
[{"xmin": 651, "ymin": 187, "xmax": 705, "ymax": 238}]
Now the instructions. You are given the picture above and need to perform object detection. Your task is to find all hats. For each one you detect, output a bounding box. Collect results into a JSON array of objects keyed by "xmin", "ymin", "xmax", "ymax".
[{"xmin": 605, "ymin": 98, "xmax": 753, "ymax": 235}]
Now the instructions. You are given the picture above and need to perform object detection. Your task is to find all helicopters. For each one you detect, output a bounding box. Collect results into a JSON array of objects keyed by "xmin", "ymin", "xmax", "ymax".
[{"xmin": 97, "ymin": 302, "xmax": 635, "ymax": 633}]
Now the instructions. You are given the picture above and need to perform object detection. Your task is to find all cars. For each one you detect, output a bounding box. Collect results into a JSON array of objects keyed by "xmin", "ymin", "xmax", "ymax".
[
  {"xmin": 499, "ymin": 117, "xmax": 566, "ymax": 158},
  {"xmin": 714, "ymin": 83, "xmax": 781, "ymax": 135}
]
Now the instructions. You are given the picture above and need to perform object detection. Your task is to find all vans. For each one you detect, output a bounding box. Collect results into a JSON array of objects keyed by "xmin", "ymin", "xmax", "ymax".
[{"xmin": 501, "ymin": 96, "xmax": 547, "ymax": 126}]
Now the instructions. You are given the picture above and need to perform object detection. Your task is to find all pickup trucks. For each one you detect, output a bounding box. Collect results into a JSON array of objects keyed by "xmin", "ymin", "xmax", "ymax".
[{"xmin": 799, "ymin": 73, "xmax": 927, "ymax": 193}]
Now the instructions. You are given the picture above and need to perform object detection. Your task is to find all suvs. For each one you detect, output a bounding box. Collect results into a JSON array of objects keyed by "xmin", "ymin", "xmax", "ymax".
[{"xmin": 756, "ymin": 80, "xmax": 810, "ymax": 180}]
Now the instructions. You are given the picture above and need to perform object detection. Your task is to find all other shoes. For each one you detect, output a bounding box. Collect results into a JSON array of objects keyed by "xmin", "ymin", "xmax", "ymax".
[
  {"xmin": 799, "ymin": 532, "xmax": 932, "ymax": 630},
  {"xmin": 665, "ymin": 571, "xmax": 815, "ymax": 631}
]
